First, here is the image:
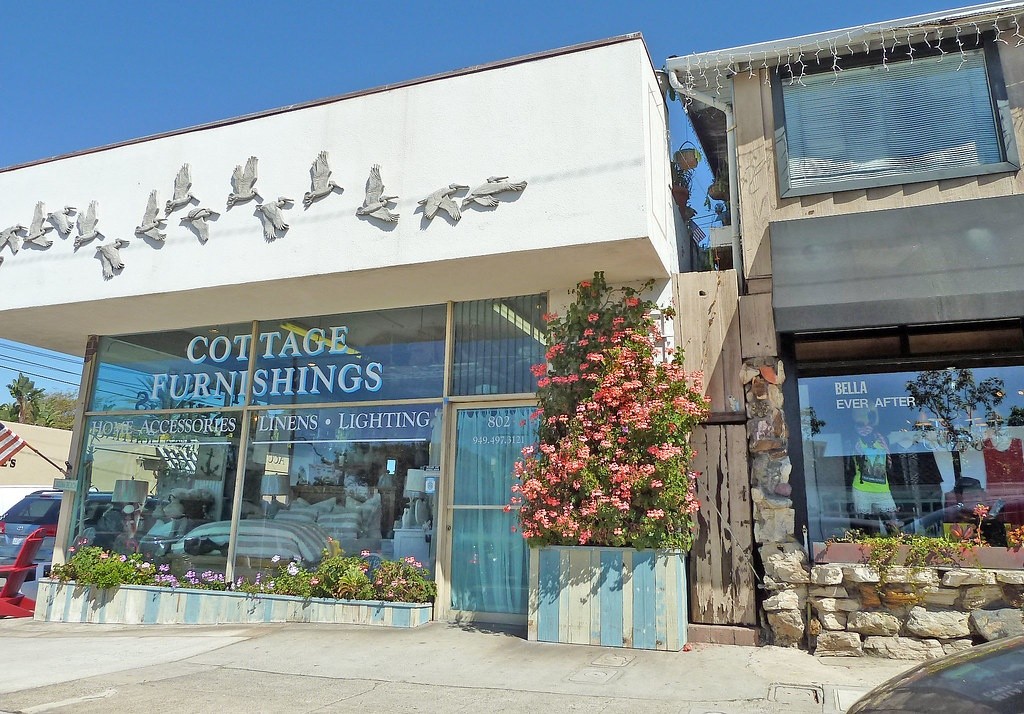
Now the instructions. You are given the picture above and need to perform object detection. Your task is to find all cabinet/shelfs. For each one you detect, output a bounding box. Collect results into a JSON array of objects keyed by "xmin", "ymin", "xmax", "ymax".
[
  {"xmin": 193, "ymin": 436, "xmax": 268, "ymax": 522},
  {"xmin": 392, "ymin": 521, "xmax": 430, "ymax": 581}
]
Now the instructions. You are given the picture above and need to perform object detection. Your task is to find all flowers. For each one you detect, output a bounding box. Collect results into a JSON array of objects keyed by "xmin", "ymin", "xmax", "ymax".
[
  {"xmin": 501, "ymin": 270, "xmax": 713, "ymax": 557},
  {"xmin": 824, "ymin": 503, "xmax": 1024, "ymax": 607},
  {"xmin": 48, "ymin": 538, "xmax": 439, "ymax": 604}
]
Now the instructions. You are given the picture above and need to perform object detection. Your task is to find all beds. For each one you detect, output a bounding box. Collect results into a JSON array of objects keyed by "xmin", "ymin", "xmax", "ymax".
[{"xmin": 172, "ymin": 484, "xmax": 398, "ymax": 584}]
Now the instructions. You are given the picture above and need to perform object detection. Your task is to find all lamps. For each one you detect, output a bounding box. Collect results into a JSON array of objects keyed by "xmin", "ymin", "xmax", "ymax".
[
  {"xmin": 111, "ymin": 477, "xmax": 148, "ymax": 539},
  {"xmin": 915, "ymin": 410, "xmax": 933, "ymax": 426},
  {"xmin": 964, "ymin": 405, "xmax": 1004, "ymax": 427},
  {"xmin": 406, "ymin": 468, "xmax": 424, "ymax": 526},
  {"xmin": 278, "ymin": 318, "xmax": 363, "ymax": 360}
]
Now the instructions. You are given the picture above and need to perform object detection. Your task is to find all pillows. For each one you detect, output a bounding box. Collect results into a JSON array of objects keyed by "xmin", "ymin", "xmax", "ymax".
[
  {"xmin": 163, "ymin": 499, "xmax": 185, "ymax": 520},
  {"xmin": 147, "ymin": 519, "xmax": 174, "ymax": 538},
  {"xmin": 274, "ymin": 508, "xmax": 319, "ymax": 523},
  {"xmin": 345, "ymin": 493, "xmax": 383, "ymax": 539},
  {"xmin": 151, "ymin": 505, "xmax": 165, "ymax": 519},
  {"xmin": 332, "ymin": 502, "xmax": 378, "ymax": 539},
  {"xmin": 297, "ymin": 497, "xmax": 337, "ymax": 509},
  {"xmin": 162, "ymin": 517, "xmax": 187, "ymax": 539},
  {"xmin": 290, "ymin": 499, "xmax": 333, "ymax": 521},
  {"xmin": 316, "ymin": 509, "xmax": 363, "ymax": 539}
]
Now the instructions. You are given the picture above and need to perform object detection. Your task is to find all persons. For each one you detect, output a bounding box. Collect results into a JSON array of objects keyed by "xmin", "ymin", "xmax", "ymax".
[{"xmin": 848, "ymin": 404, "xmax": 935, "ymax": 534}]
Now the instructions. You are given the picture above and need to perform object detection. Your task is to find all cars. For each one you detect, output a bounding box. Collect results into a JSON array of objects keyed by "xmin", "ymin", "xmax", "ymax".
[
  {"xmin": 843, "ymin": 633, "xmax": 1023, "ymax": 714},
  {"xmin": 450, "ymin": 541, "xmax": 504, "ymax": 576},
  {"xmin": 894, "ymin": 496, "xmax": 1024, "ymax": 549},
  {"xmin": 809, "ymin": 517, "xmax": 893, "ymax": 539}
]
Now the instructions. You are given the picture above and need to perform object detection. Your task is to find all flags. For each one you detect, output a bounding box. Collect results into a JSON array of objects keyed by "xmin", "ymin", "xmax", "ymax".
[{"xmin": 0, "ymin": 422, "xmax": 26, "ymax": 466}]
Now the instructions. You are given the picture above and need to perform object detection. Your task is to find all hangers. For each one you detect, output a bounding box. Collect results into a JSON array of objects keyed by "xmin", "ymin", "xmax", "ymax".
[{"xmin": 897, "ymin": 432, "xmax": 913, "ymax": 442}]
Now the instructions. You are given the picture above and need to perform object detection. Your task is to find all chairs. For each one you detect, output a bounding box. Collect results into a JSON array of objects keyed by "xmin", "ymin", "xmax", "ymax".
[{"xmin": 0, "ymin": 528, "xmax": 47, "ymax": 617}]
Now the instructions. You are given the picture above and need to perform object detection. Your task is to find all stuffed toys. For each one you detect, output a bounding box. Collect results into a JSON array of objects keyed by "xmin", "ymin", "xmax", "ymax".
[
  {"xmin": 184, "ymin": 536, "xmax": 229, "ymax": 555},
  {"xmin": 115, "ymin": 517, "xmax": 138, "ymax": 552}
]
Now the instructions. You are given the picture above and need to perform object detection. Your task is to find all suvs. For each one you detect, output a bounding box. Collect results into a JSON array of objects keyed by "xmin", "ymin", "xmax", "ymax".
[{"xmin": 0, "ymin": 487, "xmax": 169, "ymax": 578}]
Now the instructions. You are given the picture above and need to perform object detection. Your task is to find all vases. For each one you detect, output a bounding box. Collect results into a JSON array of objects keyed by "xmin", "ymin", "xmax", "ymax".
[
  {"xmin": 813, "ymin": 541, "xmax": 1024, "ymax": 570},
  {"xmin": 525, "ymin": 544, "xmax": 689, "ymax": 653}
]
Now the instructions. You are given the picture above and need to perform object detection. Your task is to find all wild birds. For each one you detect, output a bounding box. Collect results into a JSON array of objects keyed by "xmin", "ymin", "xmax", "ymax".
[{"xmin": 0, "ymin": 149, "xmax": 528, "ymax": 278}]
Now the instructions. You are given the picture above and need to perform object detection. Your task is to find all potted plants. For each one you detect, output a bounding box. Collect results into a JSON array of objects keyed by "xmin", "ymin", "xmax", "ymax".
[
  {"xmin": 703, "ymin": 182, "xmax": 729, "ymax": 212},
  {"xmin": 685, "ymin": 205, "xmax": 698, "ymax": 222},
  {"xmin": 673, "ymin": 147, "xmax": 702, "ymax": 171},
  {"xmin": 671, "ymin": 162, "xmax": 697, "ymax": 217}
]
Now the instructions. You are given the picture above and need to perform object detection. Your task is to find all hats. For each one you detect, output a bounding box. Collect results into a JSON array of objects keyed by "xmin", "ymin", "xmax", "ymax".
[{"xmin": 953, "ymin": 477, "xmax": 984, "ymax": 496}]
